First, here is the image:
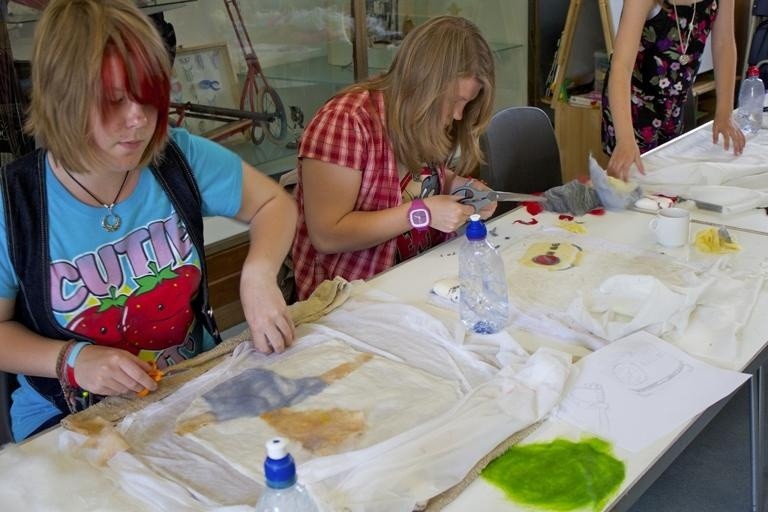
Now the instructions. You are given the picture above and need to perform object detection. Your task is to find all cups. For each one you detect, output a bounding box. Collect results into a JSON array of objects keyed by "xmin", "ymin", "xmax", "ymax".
[{"xmin": 650, "ymin": 206, "xmax": 690, "ymax": 247}]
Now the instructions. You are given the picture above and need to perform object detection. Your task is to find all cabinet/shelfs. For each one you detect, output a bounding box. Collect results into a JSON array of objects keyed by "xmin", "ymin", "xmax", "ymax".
[
  {"xmin": 553, "ymin": 78, "xmax": 741, "ymax": 190},
  {"xmin": 194, "ymin": 210, "xmax": 250, "ymax": 333}
]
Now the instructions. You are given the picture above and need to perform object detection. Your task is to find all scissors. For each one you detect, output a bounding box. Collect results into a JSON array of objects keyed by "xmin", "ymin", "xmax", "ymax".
[
  {"xmin": 449, "ymin": 185, "xmax": 548, "ymax": 215},
  {"xmin": 133, "ymin": 359, "xmax": 191, "ymax": 397}
]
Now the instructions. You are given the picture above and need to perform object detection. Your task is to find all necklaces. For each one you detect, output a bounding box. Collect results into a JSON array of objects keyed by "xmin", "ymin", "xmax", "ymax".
[
  {"xmin": 671, "ymin": 1, "xmax": 696, "ymax": 65},
  {"xmin": 57, "ymin": 160, "xmax": 130, "ymax": 232}
]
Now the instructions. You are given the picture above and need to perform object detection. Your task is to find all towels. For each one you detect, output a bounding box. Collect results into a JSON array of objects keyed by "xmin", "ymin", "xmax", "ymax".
[{"xmin": 57, "ymin": 273, "xmax": 356, "ymax": 439}]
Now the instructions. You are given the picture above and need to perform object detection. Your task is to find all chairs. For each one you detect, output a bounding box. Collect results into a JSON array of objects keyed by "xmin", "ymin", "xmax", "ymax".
[
  {"xmin": 484, "ymin": 104, "xmax": 563, "ymax": 222},
  {"xmin": 275, "ymin": 168, "xmax": 301, "ymax": 273}
]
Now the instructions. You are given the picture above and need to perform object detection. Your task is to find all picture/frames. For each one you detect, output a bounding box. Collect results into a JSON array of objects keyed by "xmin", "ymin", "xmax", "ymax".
[{"xmin": 161, "ymin": 41, "xmax": 249, "ymax": 149}]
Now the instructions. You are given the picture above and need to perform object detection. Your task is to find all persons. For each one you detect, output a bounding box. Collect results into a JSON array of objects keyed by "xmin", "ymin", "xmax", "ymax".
[
  {"xmin": 283, "ymin": 16, "xmax": 495, "ymax": 304},
  {"xmin": 0, "ymin": 0, "xmax": 297, "ymax": 444},
  {"xmin": 601, "ymin": 0, "xmax": 746, "ymax": 183}
]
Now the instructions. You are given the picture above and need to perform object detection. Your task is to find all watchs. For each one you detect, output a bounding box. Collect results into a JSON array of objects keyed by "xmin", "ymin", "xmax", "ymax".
[{"xmin": 409, "ymin": 198, "xmax": 432, "ymax": 234}]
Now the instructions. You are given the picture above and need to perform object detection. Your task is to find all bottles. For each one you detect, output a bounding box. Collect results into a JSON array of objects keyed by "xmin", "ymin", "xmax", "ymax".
[
  {"xmin": 253, "ymin": 437, "xmax": 320, "ymax": 512},
  {"xmin": 737, "ymin": 65, "xmax": 766, "ymax": 136},
  {"xmin": 458, "ymin": 214, "xmax": 511, "ymax": 335}
]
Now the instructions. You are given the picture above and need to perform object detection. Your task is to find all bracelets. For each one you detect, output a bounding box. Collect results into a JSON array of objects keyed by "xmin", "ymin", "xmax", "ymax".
[{"xmin": 57, "ymin": 338, "xmax": 92, "ymax": 388}]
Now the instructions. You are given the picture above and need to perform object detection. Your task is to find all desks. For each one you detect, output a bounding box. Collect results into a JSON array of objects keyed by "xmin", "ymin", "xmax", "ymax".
[
  {"xmin": 0, "ymin": 203, "xmax": 768, "ymax": 511},
  {"xmin": 583, "ymin": 93, "xmax": 767, "ymax": 239}
]
